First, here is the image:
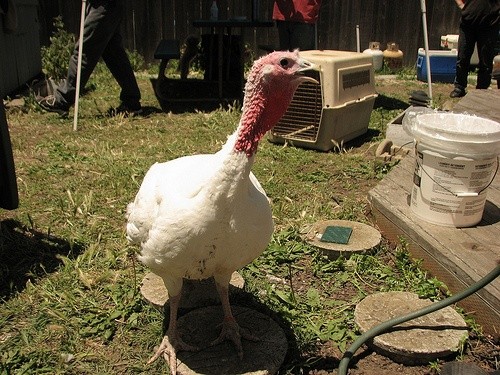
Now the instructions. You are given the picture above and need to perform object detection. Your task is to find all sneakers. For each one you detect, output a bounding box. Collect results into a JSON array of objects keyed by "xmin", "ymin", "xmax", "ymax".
[
  {"xmin": 115, "ymin": 97, "xmax": 143, "ymax": 114},
  {"xmin": 35, "ymin": 95, "xmax": 69, "ymax": 117}
]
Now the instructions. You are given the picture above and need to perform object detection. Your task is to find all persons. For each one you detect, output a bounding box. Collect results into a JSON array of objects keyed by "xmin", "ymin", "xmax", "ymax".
[
  {"xmin": 271, "ymin": 0, "xmax": 320, "ymax": 51},
  {"xmin": 34, "ymin": 0, "xmax": 142, "ymax": 117},
  {"xmin": 450, "ymin": 0, "xmax": 500, "ymax": 98}
]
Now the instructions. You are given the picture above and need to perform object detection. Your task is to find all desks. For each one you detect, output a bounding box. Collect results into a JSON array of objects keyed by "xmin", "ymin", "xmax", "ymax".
[{"xmin": 196, "ymin": 18, "xmax": 275, "ymax": 106}]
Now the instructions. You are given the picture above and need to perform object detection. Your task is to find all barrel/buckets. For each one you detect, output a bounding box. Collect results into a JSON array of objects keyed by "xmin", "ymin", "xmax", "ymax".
[{"xmin": 409, "ymin": 113, "xmax": 500, "ymax": 227}]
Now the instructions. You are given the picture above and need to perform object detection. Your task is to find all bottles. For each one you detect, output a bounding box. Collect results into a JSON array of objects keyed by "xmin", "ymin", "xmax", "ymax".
[{"xmin": 211, "ymin": 1, "xmax": 219, "ymax": 21}]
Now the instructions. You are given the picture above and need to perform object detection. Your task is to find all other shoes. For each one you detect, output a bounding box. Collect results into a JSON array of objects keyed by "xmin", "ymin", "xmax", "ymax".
[{"xmin": 450, "ymin": 84, "xmax": 466, "ymax": 98}]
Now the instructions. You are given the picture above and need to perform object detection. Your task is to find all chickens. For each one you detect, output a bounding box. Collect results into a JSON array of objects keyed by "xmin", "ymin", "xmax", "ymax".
[{"xmin": 126, "ymin": 51, "xmax": 318, "ymax": 375}]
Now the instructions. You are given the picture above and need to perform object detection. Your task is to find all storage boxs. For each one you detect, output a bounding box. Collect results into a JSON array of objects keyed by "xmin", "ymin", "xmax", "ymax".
[{"xmin": 417, "ymin": 48, "xmax": 459, "ymax": 84}]
